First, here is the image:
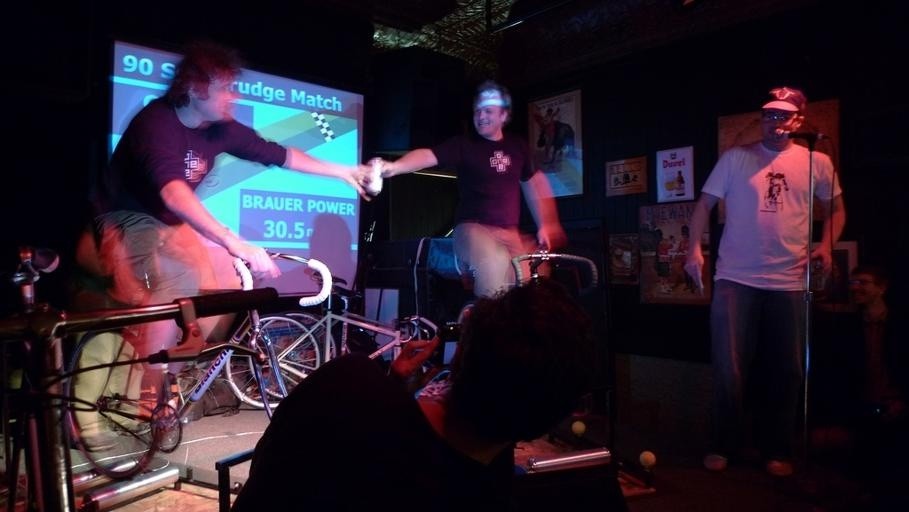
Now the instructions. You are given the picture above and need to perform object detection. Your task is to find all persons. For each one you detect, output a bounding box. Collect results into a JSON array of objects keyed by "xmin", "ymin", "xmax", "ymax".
[
  {"xmin": 368, "ymin": 78, "xmax": 568, "ymax": 308},
  {"xmin": 93, "ymin": 44, "xmax": 362, "ymax": 385},
  {"xmin": 681, "ymin": 83, "xmax": 846, "ymax": 422}
]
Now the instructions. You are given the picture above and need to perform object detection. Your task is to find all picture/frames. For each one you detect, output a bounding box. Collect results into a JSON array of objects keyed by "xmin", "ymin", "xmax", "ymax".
[{"xmin": 524, "ymin": 87, "xmax": 589, "ymax": 203}]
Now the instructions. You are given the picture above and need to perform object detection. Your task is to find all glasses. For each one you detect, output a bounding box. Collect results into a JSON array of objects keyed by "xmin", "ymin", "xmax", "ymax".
[{"xmin": 761, "ymin": 111, "xmax": 794, "ymax": 122}]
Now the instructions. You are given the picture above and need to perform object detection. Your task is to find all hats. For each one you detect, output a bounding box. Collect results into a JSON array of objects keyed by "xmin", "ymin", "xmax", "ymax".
[{"xmin": 760, "ymin": 86, "xmax": 808, "ymax": 115}]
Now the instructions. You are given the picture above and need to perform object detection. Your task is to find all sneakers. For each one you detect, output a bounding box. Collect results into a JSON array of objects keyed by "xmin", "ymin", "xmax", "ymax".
[{"xmin": 61, "ymin": 419, "xmax": 154, "ymax": 452}]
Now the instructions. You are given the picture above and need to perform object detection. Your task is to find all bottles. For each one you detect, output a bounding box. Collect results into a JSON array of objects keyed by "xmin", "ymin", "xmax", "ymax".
[{"xmin": 674, "ymin": 171, "xmax": 686, "ymax": 196}]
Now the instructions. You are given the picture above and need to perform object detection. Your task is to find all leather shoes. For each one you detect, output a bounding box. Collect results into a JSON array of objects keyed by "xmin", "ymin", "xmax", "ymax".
[
  {"xmin": 767, "ymin": 459, "xmax": 793, "ymax": 477},
  {"xmin": 703, "ymin": 454, "xmax": 727, "ymax": 471}
]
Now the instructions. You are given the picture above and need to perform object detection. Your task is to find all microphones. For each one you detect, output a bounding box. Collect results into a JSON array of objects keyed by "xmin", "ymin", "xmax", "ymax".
[{"xmin": 774, "ymin": 127, "xmax": 827, "ymax": 141}]
